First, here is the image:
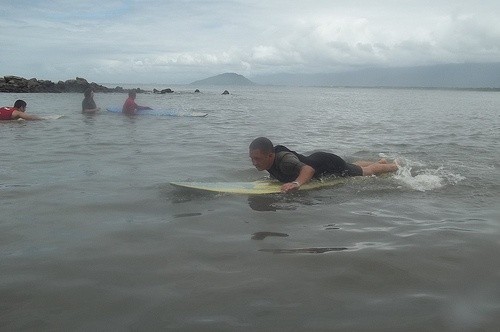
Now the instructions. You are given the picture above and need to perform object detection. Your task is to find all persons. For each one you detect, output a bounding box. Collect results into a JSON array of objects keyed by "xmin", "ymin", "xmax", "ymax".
[
  {"xmin": 122, "ymin": 90, "xmax": 153, "ymax": 113},
  {"xmin": 0, "ymin": 100, "xmax": 47, "ymax": 121},
  {"xmin": 82, "ymin": 88, "xmax": 101, "ymax": 114},
  {"xmin": 250, "ymin": 137, "xmax": 399, "ymax": 193}
]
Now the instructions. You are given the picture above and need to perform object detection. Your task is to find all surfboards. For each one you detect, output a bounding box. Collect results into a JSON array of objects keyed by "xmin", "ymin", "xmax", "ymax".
[
  {"xmin": 171, "ymin": 178, "xmax": 347, "ymax": 194},
  {"xmin": 0, "ymin": 114, "xmax": 63, "ymax": 121},
  {"xmin": 106, "ymin": 107, "xmax": 208, "ymax": 117}
]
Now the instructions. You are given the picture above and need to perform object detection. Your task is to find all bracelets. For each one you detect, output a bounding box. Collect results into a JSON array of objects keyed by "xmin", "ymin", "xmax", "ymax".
[{"xmin": 292, "ymin": 181, "xmax": 300, "ymax": 187}]
